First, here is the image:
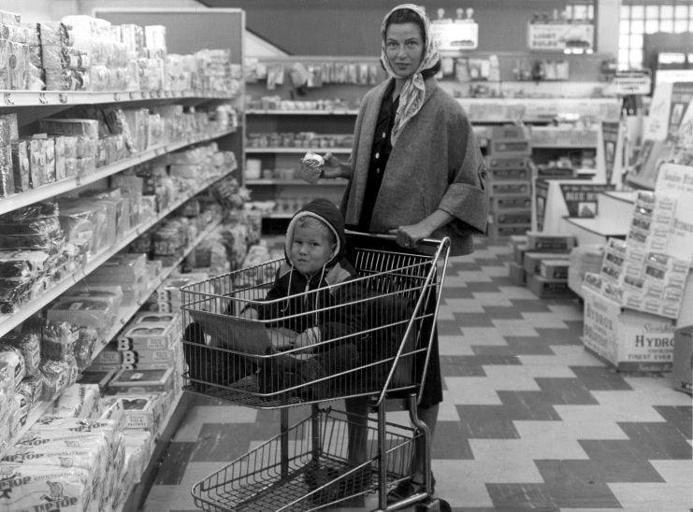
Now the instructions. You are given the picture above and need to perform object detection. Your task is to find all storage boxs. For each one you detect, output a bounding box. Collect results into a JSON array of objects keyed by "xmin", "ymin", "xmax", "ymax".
[
  {"xmin": 508, "ymin": 230, "xmax": 581, "ymax": 299},
  {"xmin": 583, "ymin": 284, "xmax": 675, "ymax": 373}
]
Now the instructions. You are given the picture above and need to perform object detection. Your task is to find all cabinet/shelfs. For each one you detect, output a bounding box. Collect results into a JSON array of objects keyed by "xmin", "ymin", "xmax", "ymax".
[
  {"xmin": 246, "ymin": 56, "xmax": 621, "ymax": 243},
  {"xmin": 0, "ymin": 88, "xmax": 239, "ymax": 512}
]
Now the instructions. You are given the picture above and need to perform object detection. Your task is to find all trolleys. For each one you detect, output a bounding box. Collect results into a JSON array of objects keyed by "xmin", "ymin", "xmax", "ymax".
[{"xmin": 179, "ymin": 228, "xmax": 452, "ymax": 512}]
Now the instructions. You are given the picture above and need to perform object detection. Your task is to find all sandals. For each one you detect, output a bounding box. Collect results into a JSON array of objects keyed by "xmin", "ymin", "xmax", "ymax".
[{"xmin": 387, "ymin": 470, "xmax": 436, "ymax": 506}]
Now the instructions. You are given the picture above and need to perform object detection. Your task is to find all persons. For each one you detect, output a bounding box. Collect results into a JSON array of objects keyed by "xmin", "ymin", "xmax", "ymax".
[
  {"xmin": 298, "ymin": 1, "xmax": 490, "ymax": 509},
  {"xmin": 181, "ymin": 197, "xmax": 371, "ymax": 405}
]
